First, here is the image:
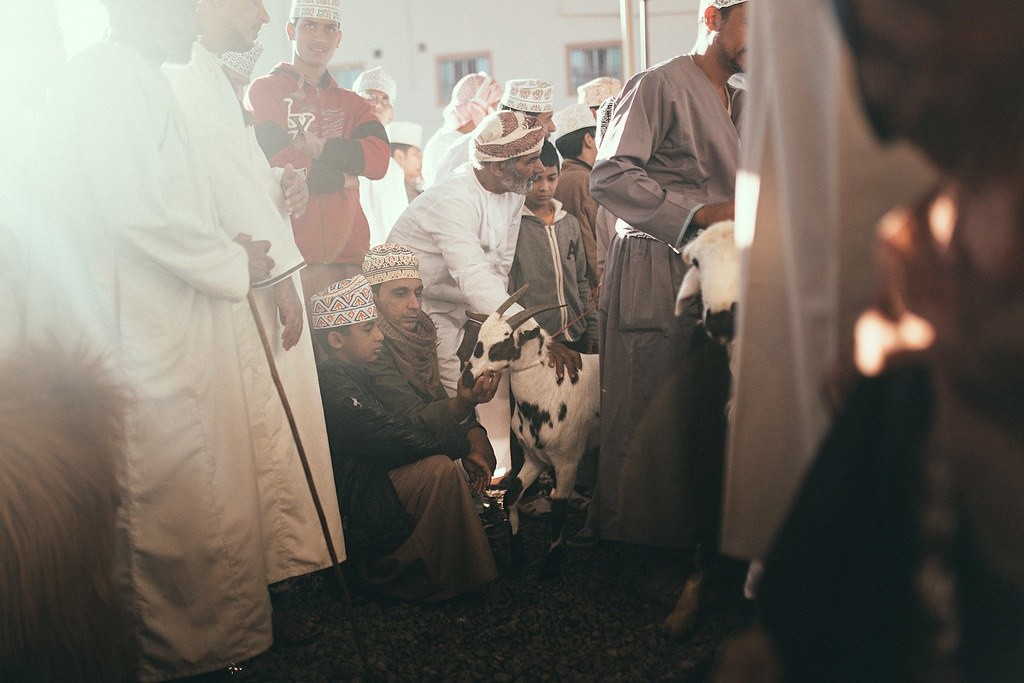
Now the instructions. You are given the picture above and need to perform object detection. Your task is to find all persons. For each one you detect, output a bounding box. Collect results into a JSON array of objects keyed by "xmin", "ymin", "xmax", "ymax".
[
  {"xmin": 0, "ymin": 0, "xmax": 346, "ymax": 683},
  {"xmin": 309, "ymin": 242, "xmax": 512, "ymax": 612},
  {"xmin": 249, "ymin": 0, "xmax": 391, "ymax": 364},
  {"xmin": 352, "ymin": 67, "xmax": 504, "ymax": 251},
  {"xmin": 719, "ymin": 0, "xmax": 1024, "ymax": 683},
  {"xmin": 387, "ymin": 77, "xmax": 622, "ymax": 547},
  {"xmin": 589, "ymin": 0, "xmax": 749, "ymax": 557}
]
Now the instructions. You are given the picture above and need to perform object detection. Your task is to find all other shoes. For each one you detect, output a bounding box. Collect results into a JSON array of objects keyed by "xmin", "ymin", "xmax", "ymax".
[
  {"xmin": 551, "ymin": 484, "xmax": 590, "ymax": 512},
  {"xmin": 515, "ymin": 490, "xmax": 551, "ymax": 516},
  {"xmin": 568, "ymin": 527, "xmax": 600, "ymax": 548}
]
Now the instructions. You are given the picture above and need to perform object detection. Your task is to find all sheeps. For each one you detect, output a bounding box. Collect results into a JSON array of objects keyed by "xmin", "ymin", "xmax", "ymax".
[
  {"xmin": 661, "ymin": 220, "xmax": 741, "ymax": 639},
  {"xmin": 459, "ymin": 281, "xmax": 603, "ymax": 583}
]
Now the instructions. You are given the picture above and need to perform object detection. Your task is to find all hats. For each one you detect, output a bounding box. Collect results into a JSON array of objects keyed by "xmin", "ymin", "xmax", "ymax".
[
  {"xmin": 353, "ymin": 67, "xmax": 397, "ymax": 102},
  {"xmin": 311, "ymin": 274, "xmax": 379, "ymax": 328},
  {"xmin": 551, "ymin": 104, "xmax": 596, "ymax": 142},
  {"xmin": 290, "ymin": 0, "xmax": 340, "ymax": 23},
  {"xmin": 221, "ymin": 39, "xmax": 263, "ymax": 76},
  {"xmin": 361, "ymin": 244, "xmax": 421, "ymax": 288},
  {"xmin": 698, "ymin": 0, "xmax": 745, "ymax": 23},
  {"xmin": 383, "ymin": 119, "xmax": 423, "ymax": 148},
  {"xmin": 577, "ymin": 77, "xmax": 621, "ymax": 107},
  {"xmin": 502, "ymin": 79, "xmax": 554, "ymax": 112}
]
